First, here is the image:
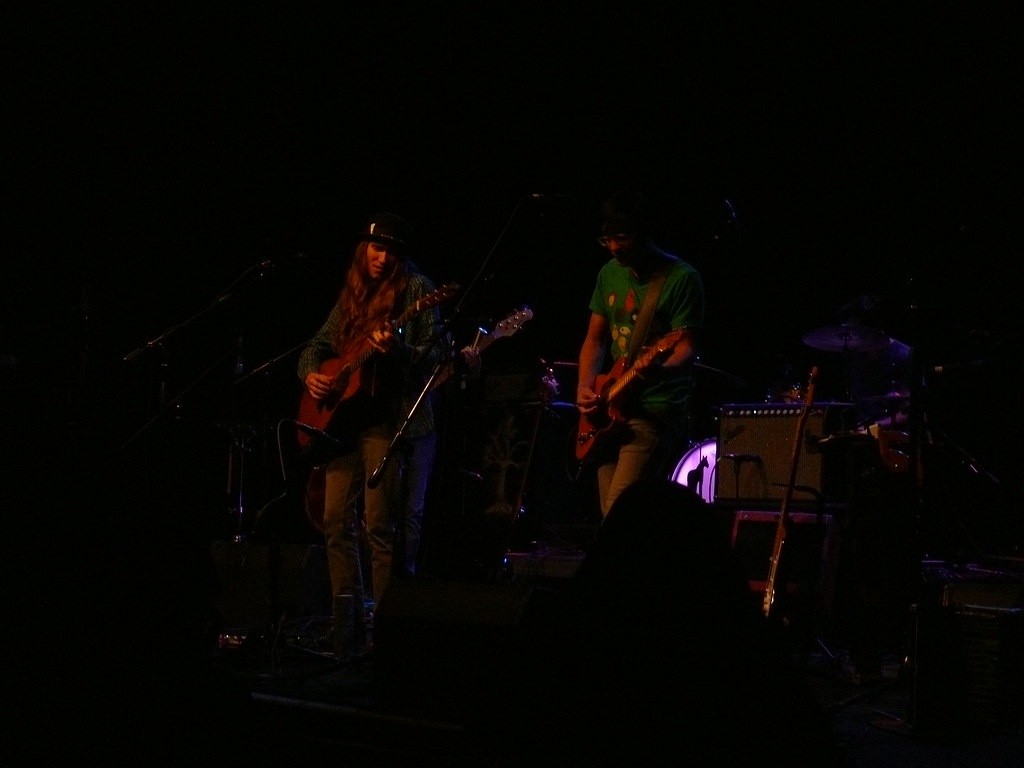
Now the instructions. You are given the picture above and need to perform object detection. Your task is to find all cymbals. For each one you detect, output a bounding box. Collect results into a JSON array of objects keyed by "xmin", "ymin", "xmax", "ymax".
[{"xmin": 803, "ymin": 326, "xmax": 888, "ymax": 355}]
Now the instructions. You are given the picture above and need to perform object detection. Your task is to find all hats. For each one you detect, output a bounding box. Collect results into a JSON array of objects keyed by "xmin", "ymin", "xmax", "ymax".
[{"xmin": 350, "ymin": 213, "xmax": 416, "ymax": 259}]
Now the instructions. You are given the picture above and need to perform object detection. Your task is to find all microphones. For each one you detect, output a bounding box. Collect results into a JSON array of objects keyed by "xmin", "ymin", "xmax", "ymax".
[
  {"xmin": 529, "ymin": 192, "xmax": 585, "ymax": 208},
  {"xmin": 721, "ymin": 454, "xmax": 760, "ymax": 464},
  {"xmin": 287, "ymin": 419, "xmax": 344, "ymax": 454},
  {"xmin": 234, "ymin": 335, "xmax": 245, "ymax": 376}
]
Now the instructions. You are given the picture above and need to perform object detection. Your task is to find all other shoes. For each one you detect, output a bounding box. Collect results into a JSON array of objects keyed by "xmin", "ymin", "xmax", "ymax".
[{"xmin": 296, "ymin": 624, "xmax": 376, "ymax": 657}]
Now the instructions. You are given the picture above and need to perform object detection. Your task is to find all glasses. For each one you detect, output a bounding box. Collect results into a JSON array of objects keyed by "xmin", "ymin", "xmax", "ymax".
[{"xmin": 597, "ymin": 231, "xmax": 629, "ymax": 246}]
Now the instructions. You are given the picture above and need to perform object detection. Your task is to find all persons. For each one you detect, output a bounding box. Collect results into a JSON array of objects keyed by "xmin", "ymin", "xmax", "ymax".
[
  {"xmin": 298, "ymin": 213, "xmax": 451, "ymax": 653},
  {"xmin": 576, "ymin": 207, "xmax": 704, "ymax": 519},
  {"xmin": 393, "ymin": 343, "xmax": 484, "ymax": 576},
  {"xmin": 828, "ymin": 298, "xmax": 922, "ymax": 450}
]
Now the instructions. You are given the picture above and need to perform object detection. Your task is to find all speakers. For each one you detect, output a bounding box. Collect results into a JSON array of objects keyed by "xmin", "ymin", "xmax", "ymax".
[
  {"xmin": 717, "ymin": 403, "xmax": 858, "ymax": 503},
  {"xmin": 208, "ymin": 541, "xmax": 333, "ymax": 620},
  {"xmin": 372, "ymin": 576, "xmax": 594, "ymax": 689}
]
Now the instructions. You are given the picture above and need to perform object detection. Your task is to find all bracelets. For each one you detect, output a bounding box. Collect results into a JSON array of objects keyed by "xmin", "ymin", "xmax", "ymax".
[{"xmin": 890, "ymin": 414, "xmax": 896, "ymax": 426}]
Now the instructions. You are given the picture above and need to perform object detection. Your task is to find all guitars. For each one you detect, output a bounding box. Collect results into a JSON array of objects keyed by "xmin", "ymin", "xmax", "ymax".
[
  {"xmin": 291, "ymin": 283, "xmax": 458, "ymax": 448},
  {"xmin": 573, "ymin": 323, "xmax": 695, "ymax": 462}
]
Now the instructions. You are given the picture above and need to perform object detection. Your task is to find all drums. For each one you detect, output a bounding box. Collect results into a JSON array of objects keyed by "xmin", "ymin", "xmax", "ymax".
[{"xmin": 667, "ymin": 438, "xmax": 716, "ymax": 504}]
